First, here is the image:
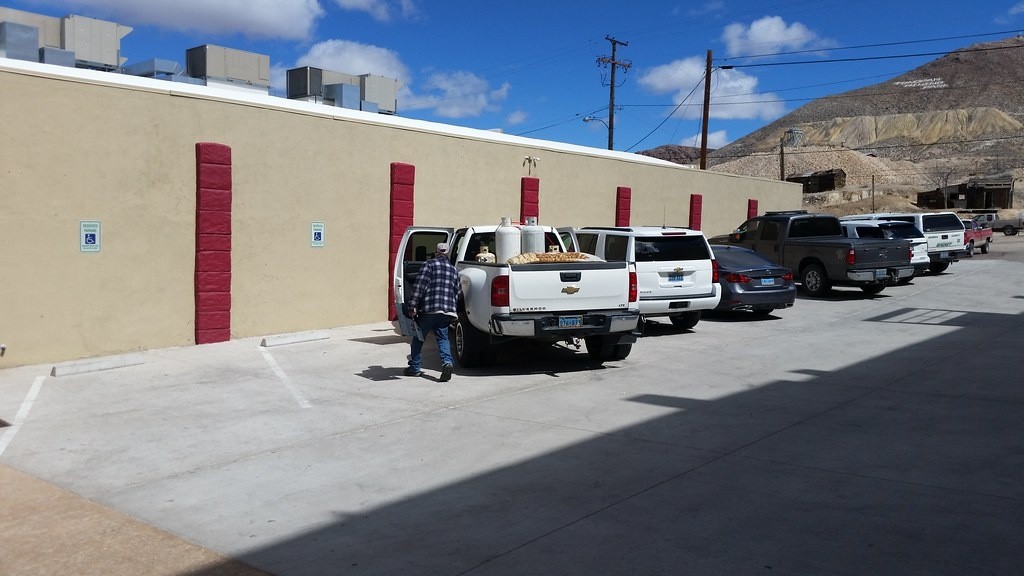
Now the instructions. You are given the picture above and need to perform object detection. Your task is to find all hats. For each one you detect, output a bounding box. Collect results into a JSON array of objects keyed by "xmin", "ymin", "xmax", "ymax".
[{"xmin": 436, "ymin": 243, "xmax": 449, "ymax": 254}]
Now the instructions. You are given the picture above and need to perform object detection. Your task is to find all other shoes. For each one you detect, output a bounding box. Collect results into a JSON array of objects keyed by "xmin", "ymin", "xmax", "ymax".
[
  {"xmin": 404, "ymin": 367, "xmax": 421, "ymax": 377},
  {"xmin": 439, "ymin": 364, "xmax": 453, "ymax": 382}
]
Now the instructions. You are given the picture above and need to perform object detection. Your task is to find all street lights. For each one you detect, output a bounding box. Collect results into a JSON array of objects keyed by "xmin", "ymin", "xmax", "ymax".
[{"xmin": 583, "ymin": 116, "xmax": 632, "ymax": 150}]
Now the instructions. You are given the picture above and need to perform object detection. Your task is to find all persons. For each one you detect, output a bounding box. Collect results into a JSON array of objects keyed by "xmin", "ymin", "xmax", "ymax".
[{"xmin": 404, "ymin": 252, "xmax": 464, "ymax": 382}]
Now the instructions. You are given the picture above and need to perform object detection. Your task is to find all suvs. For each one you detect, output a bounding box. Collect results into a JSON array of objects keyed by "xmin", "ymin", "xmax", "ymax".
[
  {"xmin": 838, "ymin": 212, "xmax": 966, "ymax": 275},
  {"xmin": 561, "ymin": 226, "xmax": 722, "ymax": 329},
  {"xmin": 839, "ymin": 220, "xmax": 931, "ymax": 285}
]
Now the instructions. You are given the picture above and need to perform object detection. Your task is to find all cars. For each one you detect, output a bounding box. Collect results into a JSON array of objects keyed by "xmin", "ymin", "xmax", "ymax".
[{"xmin": 700, "ymin": 245, "xmax": 796, "ymax": 317}]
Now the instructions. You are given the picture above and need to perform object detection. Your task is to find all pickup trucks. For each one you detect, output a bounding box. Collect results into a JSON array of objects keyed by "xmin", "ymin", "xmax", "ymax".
[
  {"xmin": 707, "ymin": 209, "xmax": 915, "ymax": 296},
  {"xmin": 393, "ymin": 225, "xmax": 641, "ymax": 361},
  {"xmin": 959, "ymin": 218, "xmax": 994, "ymax": 258},
  {"xmin": 971, "ymin": 213, "xmax": 1024, "ymax": 235}
]
